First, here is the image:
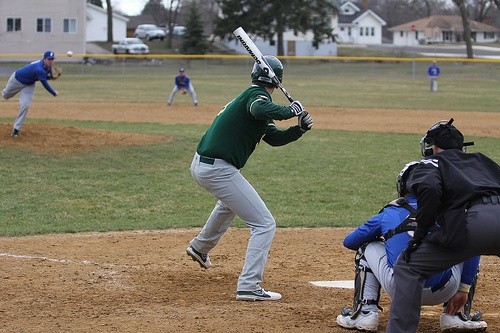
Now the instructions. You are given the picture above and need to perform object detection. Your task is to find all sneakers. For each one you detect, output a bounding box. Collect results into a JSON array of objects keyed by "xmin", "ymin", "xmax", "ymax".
[
  {"xmin": 336, "ymin": 310, "xmax": 380, "ymax": 331},
  {"xmin": 439, "ymin": 313, "xmax": 488, "ymax": 333},
  {"xmin": 186, "ymin": 245, "xmax": 212, "ymax": 269},
  {"xmin": 236, "ymin": 288, "xmax": 282, "ymax": 301}
]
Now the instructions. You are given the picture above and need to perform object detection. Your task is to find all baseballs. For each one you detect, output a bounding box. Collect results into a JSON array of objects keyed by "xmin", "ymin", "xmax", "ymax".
[{"xmin": 66, "ymin": 50, "xmax": 73, "ymax": 58}]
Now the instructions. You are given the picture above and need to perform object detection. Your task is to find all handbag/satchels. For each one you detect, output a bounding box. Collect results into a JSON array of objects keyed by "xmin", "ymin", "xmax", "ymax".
[{"xmin": 444, "ymin": 208, "xmax": 468, "ymax": 249}]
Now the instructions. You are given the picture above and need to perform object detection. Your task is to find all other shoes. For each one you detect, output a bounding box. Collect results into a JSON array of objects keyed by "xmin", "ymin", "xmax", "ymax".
[
  {"xmin": 11, "ymin": 129, "xmax": 20, "ymax": 137},
  {"xmin": 195, "ymin": 102, "xmax": 198, "ymax": 106},
  {"xmin": 168, "ymin": 102, "xmax": 171, "ymax": 106}
]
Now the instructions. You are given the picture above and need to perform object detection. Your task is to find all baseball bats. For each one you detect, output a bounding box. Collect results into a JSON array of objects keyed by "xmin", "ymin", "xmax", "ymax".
[{"xmin": 232, "ymin": 26, "xmax": 294, "ymax": 103}]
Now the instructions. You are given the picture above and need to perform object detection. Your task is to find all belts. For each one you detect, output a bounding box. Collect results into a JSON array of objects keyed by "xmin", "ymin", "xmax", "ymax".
[
  {"xmin": 200, "ymin": 157, "xmax": 215, "ymax": 165},
  {"xmin": 467, "ymin": 195, "xmax": 500, "ymax": 209},
  {"xmin": 431, "ymin": 269, "xmax": 453, "ymax": 293}
]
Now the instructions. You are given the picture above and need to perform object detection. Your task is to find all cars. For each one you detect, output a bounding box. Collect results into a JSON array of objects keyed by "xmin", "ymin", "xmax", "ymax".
[
  {"xmin": 165, "ymin": 26, "xmax": 186, "ymax": 37},
  {"xmin": 134, "ymin": 24, "xmax": 166, "ymax": 41},
  {"xmin": 111, "ymin": 38, "xmax": 150, "ymax": 54}
]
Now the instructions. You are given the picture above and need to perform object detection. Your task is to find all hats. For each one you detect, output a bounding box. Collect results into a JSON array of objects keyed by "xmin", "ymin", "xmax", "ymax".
[
  {"xmin": 179, "ymin": 68, "xmax": 185, "ymax": 72},
  {"xmin": 44, "ymin": 51, "xmax": 55, "ymax": 59},
  {"xmin": 424, "ymin": 120, "xmax": 464, "ymax": 151}
]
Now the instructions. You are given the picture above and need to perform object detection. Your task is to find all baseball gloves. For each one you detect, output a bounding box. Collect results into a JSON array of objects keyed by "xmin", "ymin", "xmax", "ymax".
[
  {"xmin": 49, "ymin": 63, "xmax": 63, "ymax": 81},
  {"xmin": 179, "ymin": 90, "xmax": 188, "ymax": 95}
]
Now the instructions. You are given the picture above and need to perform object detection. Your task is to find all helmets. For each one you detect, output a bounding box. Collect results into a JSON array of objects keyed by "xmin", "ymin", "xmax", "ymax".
[{"xmin": 250, "ymin": 55, "xmax": 283, "ymax": 89}]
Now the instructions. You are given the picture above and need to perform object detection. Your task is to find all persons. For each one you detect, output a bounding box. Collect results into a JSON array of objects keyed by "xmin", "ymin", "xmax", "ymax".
[
  {"xmin": 186, "ymin": 54, "xmax": 314, "ymax": 301},
  {"xmin": 427, "ymin": 60, "xmax": 440, "ymax": 91},
  {"xmin": 337, "ymin": 160, "xmax": 488, "ymax": 333},
  {"xmin": 167, "ymin": 67, "xmax": 199, "ymax": 105},
  {"xmin": 387, "ymin": 118, "xmax": 500, "ymax": 333},
  {"xmin": 1, "ymin": 51, "xmax": 60, "ymax": 136}
]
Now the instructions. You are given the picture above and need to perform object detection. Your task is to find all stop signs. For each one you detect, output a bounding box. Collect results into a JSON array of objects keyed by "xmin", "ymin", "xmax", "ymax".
[{"xmin": 411, "ymin": 24, "xmax": 417, "ymax": 32}]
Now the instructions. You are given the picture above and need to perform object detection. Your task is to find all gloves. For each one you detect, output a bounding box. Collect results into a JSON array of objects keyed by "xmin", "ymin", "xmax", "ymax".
[
  {"xmin": 297, "ymin": 112, "xmax": 314, "ymax": 133},
  {"xmin": 289, "ymin": 100, "xmax": 305, "ymax": 116}
]
